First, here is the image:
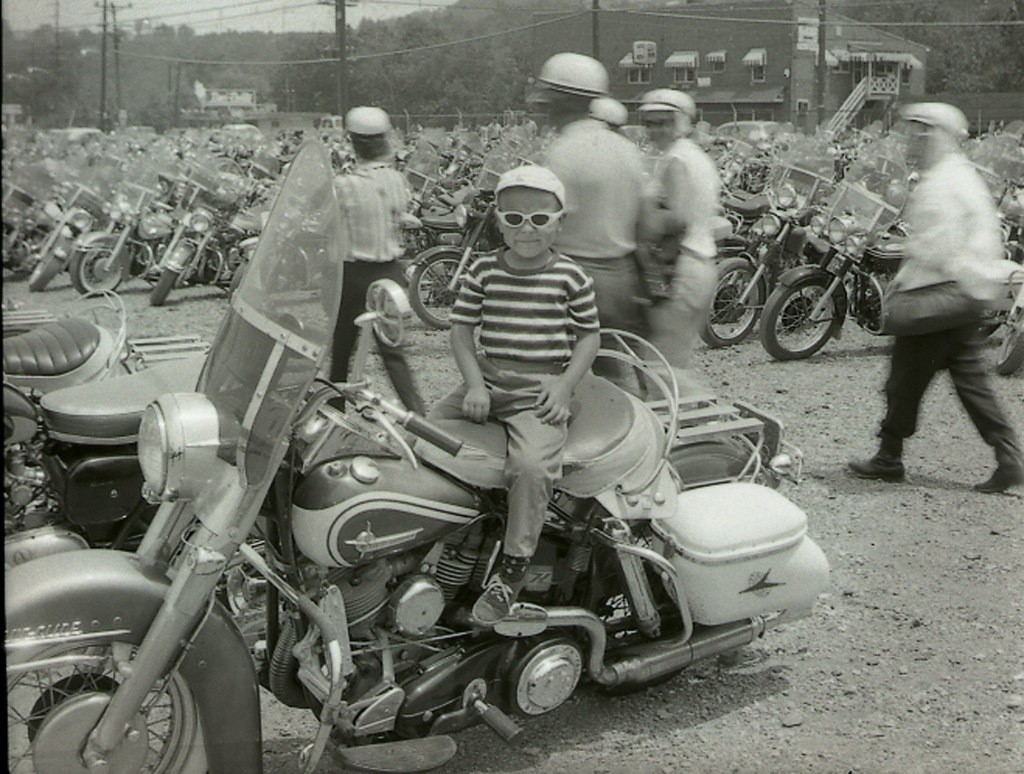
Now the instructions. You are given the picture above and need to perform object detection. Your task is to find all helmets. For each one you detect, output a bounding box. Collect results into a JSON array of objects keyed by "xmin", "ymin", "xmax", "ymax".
[
  {"xmin": 637, "ymin": 88, "xmax": 696, "ymax": 122},
  {"xmin": 345, "ymin": 106, "xmax": 391, "ymax": 135},
  {"xmin": 588, "ymin": 97, "xmax": 629, "ymax": 125},
  {"xmin": 537, "ymin": 52, "xmax": 609, "ymax": 98}
]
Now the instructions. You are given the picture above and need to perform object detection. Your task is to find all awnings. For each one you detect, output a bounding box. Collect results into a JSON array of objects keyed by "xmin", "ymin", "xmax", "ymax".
[
  {"xmin": 665, "ymin": 51, "xmax": 700, "ymax": 68},
  {"xmin": 706, "ymin": 51, "xmax": 727, "ymax": 63},
  {"xmin": 619, "ymin": 52, "xmax": 653, "ymax": 69},
  {"xmin": 846, "ymin": 40, "xmax": 923, "ymax": 70},
  {"xmin": 743, "ymin": 48, "xmax": 767, "ymax": 67}
]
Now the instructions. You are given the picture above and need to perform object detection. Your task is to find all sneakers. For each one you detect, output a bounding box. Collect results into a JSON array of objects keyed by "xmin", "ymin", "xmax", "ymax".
[{"xmin": 472, "ymin": 569, "xmax": 529, "ymax": 627}]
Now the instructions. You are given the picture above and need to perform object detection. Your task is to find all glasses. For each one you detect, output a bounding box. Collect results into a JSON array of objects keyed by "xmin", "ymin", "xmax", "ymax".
[
  {"xmin": 495, "ymin": 207, "xmax": 564, "ymax": 228},
  {"xmin": 644, "ymin": 119, "xmax": 674, "ymax": 128}
]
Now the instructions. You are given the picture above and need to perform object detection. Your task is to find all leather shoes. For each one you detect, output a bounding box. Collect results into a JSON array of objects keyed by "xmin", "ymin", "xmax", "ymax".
[
  {"xmin": 974, "ymin": 475, "xmax": 1012, "ymax": 493},
  {"xmin": 849, "ymin": 451, "xmax": 904, "ymax": 483}
]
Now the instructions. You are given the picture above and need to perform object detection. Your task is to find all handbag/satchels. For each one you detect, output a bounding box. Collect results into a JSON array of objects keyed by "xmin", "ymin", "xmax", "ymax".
[{"xmin": 879, "ymin": 279, "xmax": 981, "ymax": 336}]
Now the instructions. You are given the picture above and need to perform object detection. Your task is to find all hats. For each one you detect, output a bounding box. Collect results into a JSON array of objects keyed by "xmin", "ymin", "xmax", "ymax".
[
  {"xmin": 903, "ymin": 102, "xmax": 970, "ymax": 143},
  {"xmin": 494, "ymin": 166, "xmax": 565, "ymax": 208}
]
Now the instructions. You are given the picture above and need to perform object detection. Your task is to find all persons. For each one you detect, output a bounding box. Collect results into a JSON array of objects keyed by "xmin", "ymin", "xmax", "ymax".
[
  {"xmin": 532, "ymin": 49, "xmax": 722, "ymax": 377},
  {"xmin": 327, "ymin": 104, "xmax": 428, "ymax": 417},
  {"xmin": 847, "ymin": 103, "xmax": 1024, "ymax": 494},
  {"xmin": 424, "ymin": 162, "xmax": 602, "ymax": 625}
]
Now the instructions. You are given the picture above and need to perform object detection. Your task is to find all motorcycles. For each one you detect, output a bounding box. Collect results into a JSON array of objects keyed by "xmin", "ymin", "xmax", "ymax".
[
  {"xmin": 0, "ymin": 137, "xmax": 833, "ymax": 774},
  {"xmin": 1, "ymin": 93, "xmax": 1024, "ymax": 664}
]
